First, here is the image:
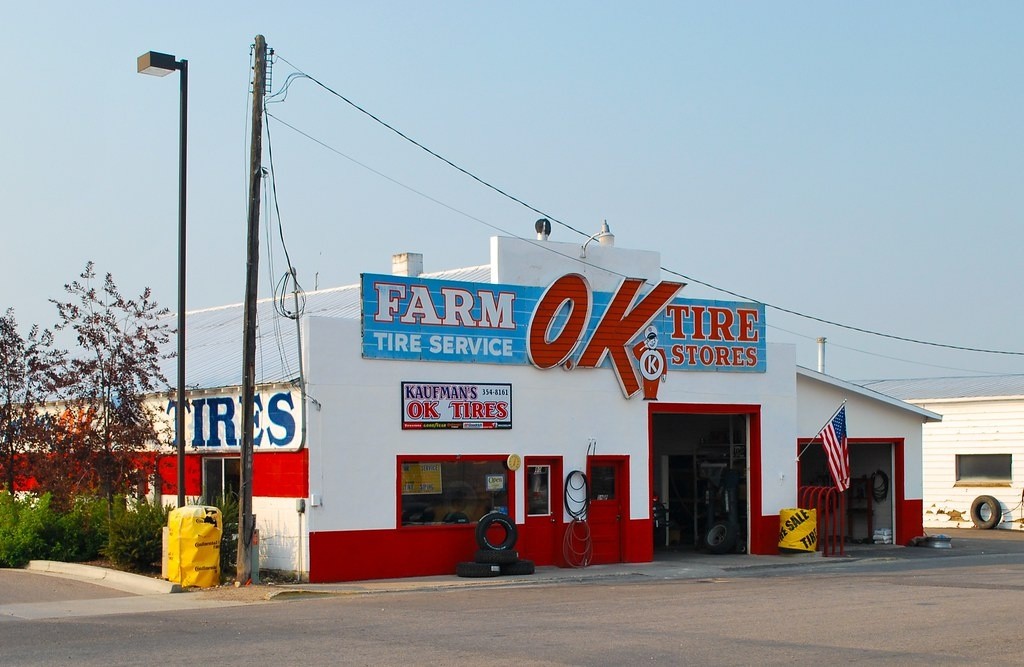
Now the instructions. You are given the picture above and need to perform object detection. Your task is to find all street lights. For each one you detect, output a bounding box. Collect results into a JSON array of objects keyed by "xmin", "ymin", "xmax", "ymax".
[{"xmin": 138, "ymin": 51, "xmax": 189, "ymax": 508}]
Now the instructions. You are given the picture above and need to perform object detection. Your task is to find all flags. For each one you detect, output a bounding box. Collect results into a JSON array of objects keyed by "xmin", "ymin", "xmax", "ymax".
[{"xmin": 820, "ymin": 404, "xmax": 852, "ymax": 494}]
